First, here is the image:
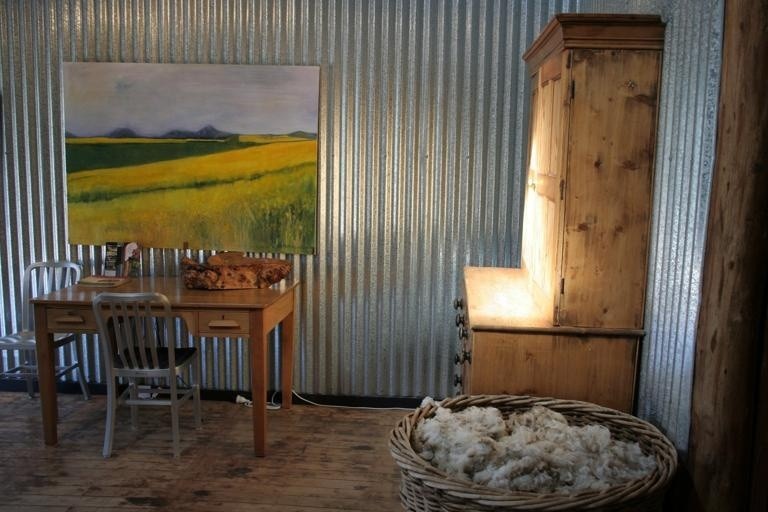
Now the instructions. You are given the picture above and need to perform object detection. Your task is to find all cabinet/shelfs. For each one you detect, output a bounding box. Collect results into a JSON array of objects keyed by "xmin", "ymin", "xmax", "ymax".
[{"xmin": 448, "ymin": 13, "xmax": 668, "ymax": 415}]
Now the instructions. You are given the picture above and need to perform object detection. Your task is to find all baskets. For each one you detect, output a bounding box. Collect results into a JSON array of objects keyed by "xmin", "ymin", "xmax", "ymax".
[{"xmin": 388, "ymin": 394, "xmax": 678, "ymax": 512}]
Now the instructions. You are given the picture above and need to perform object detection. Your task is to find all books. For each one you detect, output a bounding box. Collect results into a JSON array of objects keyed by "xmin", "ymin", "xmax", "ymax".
[
  {"xmin": 101, "ymin": 241, "xmax": 142, "ymax": 279},
  {"xmin": 75, "ymin": 274, "xmax": 131, "ymax": 288}
]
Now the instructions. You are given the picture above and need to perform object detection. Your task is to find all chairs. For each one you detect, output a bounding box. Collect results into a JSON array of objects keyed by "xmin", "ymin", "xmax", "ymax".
[
  {"xmin": 90, "ymin": 293, "xmax": 203, "ymax": 464},
  {"xmin": 1, "ymin": 263, "xmax": 88, "ymax": 407}
]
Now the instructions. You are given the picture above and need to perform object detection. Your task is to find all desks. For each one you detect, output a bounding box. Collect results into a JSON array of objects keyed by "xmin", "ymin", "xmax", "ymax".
[{"xmin": 27, "ymin": 274, "xmax": 304, "ymax": 458}]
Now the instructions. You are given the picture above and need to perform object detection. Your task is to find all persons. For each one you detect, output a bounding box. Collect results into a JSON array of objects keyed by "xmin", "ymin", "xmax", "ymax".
[{"xmin": 124, "ymin": 241, "xmax": 138, "ymax": 278}]
